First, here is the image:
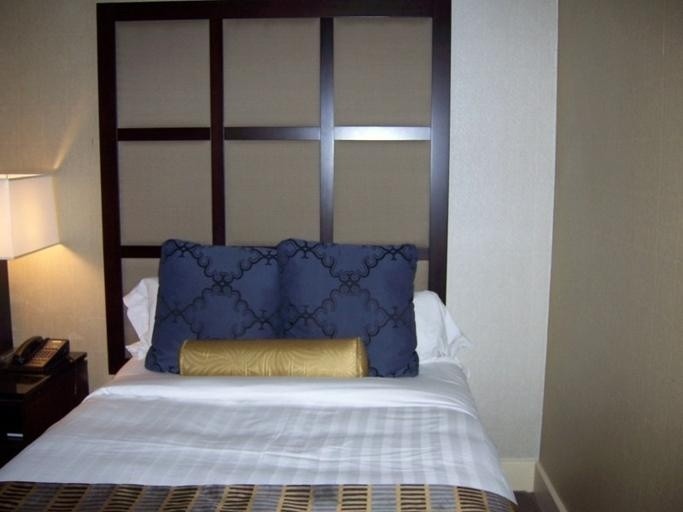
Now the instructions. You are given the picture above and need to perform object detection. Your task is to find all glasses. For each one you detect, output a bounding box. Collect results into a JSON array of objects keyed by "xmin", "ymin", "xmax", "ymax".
[{"xmin": 0, "ymin": 173, "xmax": 59, "ymax": 356}]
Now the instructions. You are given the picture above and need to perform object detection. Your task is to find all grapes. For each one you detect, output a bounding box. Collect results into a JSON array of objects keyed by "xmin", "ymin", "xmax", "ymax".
[{"xmin": 0, "ymin": 351, "xmax": 90, "ymax": 469}]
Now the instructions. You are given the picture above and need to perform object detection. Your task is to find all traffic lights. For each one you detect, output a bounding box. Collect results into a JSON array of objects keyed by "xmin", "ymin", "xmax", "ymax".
[
  {"xmin": 179, "ymin": 336, "xmax": 368, "ymax": 378},
  {"xmin": 276, "ymin": 238, "xmax": 419, "ymax": 378},
  {"xmin": 144, "ymin": 238, "xmax": 279, "ymax": 375}
]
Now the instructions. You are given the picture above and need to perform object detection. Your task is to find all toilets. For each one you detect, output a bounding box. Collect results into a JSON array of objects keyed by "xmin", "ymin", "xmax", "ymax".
[{"xmin": 12, "ymin": 335, "xmax": 69, "ymax": 372}]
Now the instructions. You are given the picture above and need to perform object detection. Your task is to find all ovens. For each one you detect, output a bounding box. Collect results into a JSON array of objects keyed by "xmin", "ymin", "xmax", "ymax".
[{"xmin": 0, "ymin": 352, "xmax": 522, "ymax": 511}]
